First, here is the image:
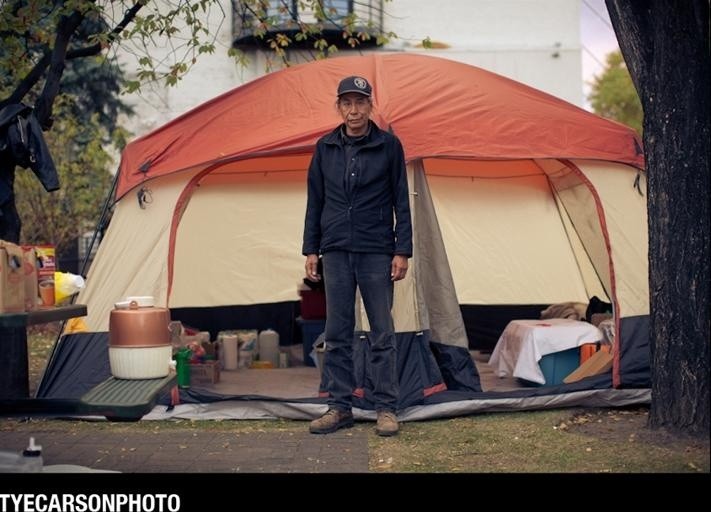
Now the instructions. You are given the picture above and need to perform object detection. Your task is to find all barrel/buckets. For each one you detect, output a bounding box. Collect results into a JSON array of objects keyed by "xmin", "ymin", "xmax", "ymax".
[{"xmin": 109, "ymin": 306, "xmax": 177, "ymax": 378}]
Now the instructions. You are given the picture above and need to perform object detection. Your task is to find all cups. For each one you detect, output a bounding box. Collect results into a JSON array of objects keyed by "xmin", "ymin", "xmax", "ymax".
[{"xmin": 39, "ymin": 281, "xmax": 55, "ymax": 306}]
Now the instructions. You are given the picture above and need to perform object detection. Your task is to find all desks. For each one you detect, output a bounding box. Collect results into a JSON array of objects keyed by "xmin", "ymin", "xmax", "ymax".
[{"xmin": 0, "ymin": 304, "xmax": 88, "ymax": 416}]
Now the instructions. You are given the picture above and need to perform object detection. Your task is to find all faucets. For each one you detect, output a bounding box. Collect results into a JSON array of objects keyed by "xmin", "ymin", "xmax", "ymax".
[{"xmin": 170, "ymin": 358, "xmax": 177, "ymax": 371}]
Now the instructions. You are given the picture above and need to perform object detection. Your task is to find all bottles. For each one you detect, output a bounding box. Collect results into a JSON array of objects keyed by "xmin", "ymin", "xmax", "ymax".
[
  {"xmin": 19, "ymin": 437, "xmax": 44, "ymax": 474},
  {"xmin": 225, "ymin": 334, "xmax": 238, "ymax": 372},
  {"xmin": 175, "ymin": 344, "xmax": 191, "ymax": 388}
]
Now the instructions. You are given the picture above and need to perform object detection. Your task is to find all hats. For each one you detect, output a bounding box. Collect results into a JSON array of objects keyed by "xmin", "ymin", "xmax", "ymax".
[{"xmin": 337, "ymin": 76, "xmax": 372, "ymax": 98}]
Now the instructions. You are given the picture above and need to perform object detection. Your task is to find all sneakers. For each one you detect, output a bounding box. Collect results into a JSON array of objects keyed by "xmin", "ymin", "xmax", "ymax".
[
  {"xmin": 308, "ymin": 407, "xmax": 354, "ymax": 434},
  {"xmin": 376, "ymin": 410, "xmax": 399, "ymax": 437}
]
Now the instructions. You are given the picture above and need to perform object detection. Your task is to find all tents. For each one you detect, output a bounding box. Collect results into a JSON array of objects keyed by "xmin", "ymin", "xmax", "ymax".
[{"xmin": 32, "ymin": 53, "xmax": 652, "ymax": 421}]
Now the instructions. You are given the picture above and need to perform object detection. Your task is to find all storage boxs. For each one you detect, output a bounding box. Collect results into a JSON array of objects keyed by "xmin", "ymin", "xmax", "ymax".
[
  {"xmin": 189, "ymin": 359, "xmax": 220, "ymax": 383},
  {"xmin": 293, "ymin": 281, "xmax": 327, "ymax": 368}
]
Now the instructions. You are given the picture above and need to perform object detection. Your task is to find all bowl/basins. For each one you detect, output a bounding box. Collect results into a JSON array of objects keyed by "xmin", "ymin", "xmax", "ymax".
[{"xmin": 109, "ymin": 307, "xmax": 172, "ymax": 379}]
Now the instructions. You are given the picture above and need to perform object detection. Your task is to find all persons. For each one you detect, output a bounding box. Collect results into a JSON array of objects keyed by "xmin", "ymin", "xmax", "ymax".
[{"xmin": 303, "ymin": 76, "xmax": 413, "ymax": 435}]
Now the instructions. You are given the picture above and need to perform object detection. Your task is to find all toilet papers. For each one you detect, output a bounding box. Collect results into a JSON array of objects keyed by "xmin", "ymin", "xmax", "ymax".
[{"xmin": 221, "ymin": 335, "xmax": 240, "ymax": 372}]
